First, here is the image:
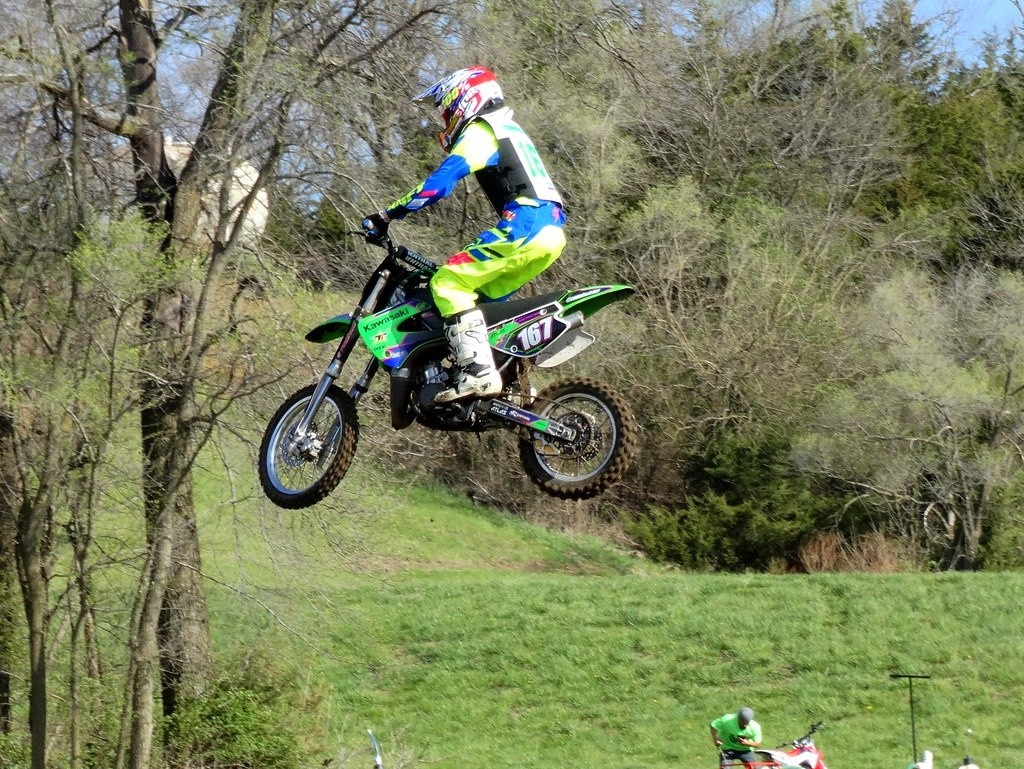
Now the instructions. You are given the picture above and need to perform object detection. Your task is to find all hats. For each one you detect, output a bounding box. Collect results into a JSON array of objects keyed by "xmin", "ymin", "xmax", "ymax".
[{"xmin": 739, "ymin": 708, "xmax": 752, "ymax": 722}]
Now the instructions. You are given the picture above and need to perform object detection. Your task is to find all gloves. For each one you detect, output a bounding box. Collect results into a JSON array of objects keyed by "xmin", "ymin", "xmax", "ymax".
[{"xmin": 362, "ymin": 210, "xmax": 388, "ymax": 241}]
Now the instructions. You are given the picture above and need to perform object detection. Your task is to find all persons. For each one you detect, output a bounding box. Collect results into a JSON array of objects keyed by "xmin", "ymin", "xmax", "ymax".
[
  {"xmin": 362, "ymin": 65, "xmax": 566, "ymax": 403},
  {"xmin": 710, "ymin": 707, "xmax": 763, "ymax": 769}
]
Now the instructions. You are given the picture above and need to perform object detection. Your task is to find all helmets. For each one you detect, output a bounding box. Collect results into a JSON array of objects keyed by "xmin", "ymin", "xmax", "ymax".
[{"xmin": 411, "ymin": 65, "xmax": 503, "ymax": 153}]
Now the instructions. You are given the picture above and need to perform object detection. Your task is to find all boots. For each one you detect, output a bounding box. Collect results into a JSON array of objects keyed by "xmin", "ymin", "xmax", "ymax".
[{"xmin": 434, "ymin": 308, "xmax": 503, "ymax": 403}]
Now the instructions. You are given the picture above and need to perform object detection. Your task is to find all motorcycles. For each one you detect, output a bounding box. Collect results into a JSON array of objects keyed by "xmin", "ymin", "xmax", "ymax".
[
  {"xmin": 754, "ymin": 720, "xmax": 828, "ymax": 769},
  {"xmin": 258, "ymin": 211, "xmax": 637, "ymax": 510}
]
